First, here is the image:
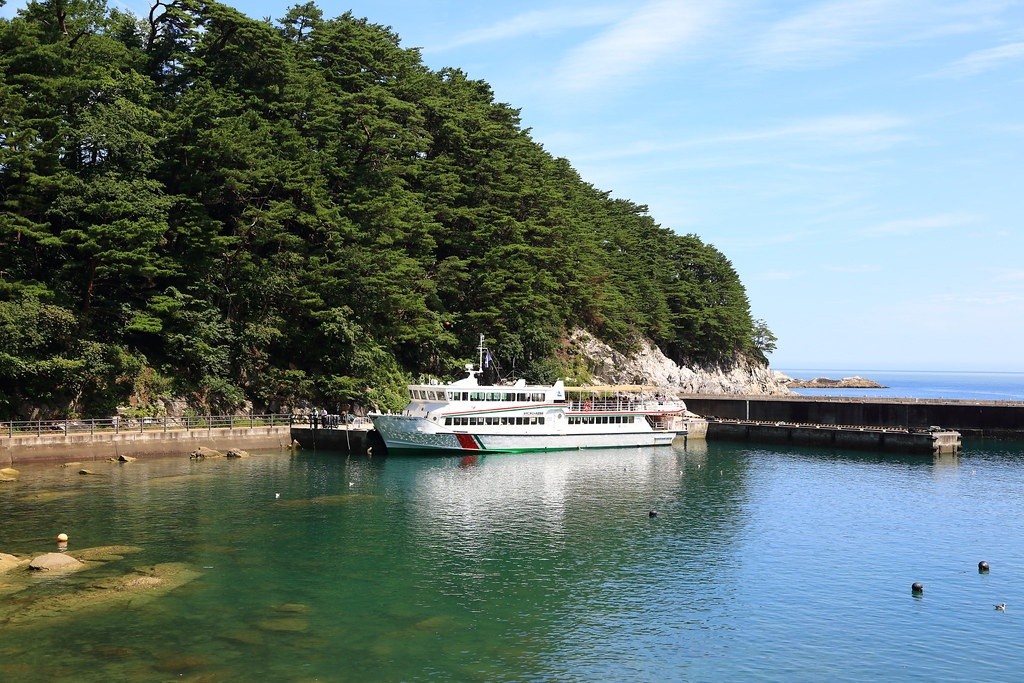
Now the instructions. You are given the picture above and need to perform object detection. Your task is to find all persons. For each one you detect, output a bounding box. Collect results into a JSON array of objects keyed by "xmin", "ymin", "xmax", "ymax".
[
  {"xmin": 582, "ymin": 395, "xmax": 638, "ymax": 410},
  {"xmin": 320, "ymin": 408, "xmax": 327, "ymax": 428},
  {"xmin": 655, "ymin": 392, "xmax": 661, "ymax": 400},
  {"xmin": 312, "ymin": 407, "xmax": 319, "ymax": 429}
]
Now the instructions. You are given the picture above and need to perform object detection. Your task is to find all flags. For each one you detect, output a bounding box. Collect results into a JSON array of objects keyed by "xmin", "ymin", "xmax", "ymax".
[{"xmin": 485, "ymin": 351, "xmax": 492, "ymax": 368}]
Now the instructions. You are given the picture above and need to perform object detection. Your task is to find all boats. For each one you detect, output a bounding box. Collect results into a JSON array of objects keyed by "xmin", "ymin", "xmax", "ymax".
[{"xmin": 367, "ymin": 334, "xmax": 690, "ymax": 453}]
